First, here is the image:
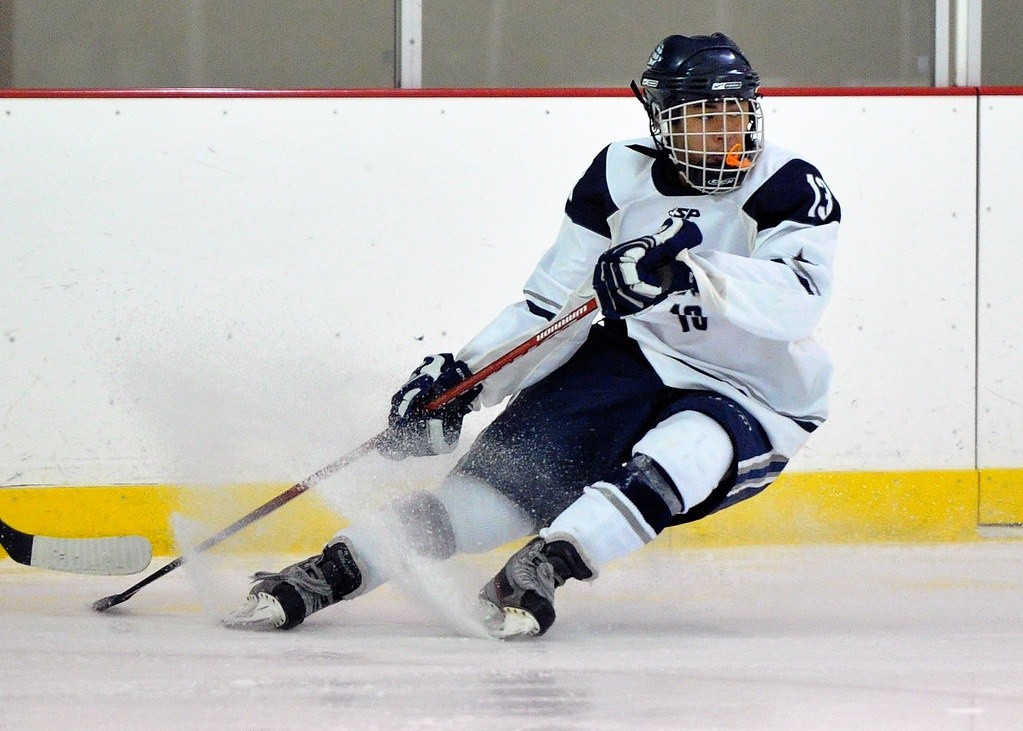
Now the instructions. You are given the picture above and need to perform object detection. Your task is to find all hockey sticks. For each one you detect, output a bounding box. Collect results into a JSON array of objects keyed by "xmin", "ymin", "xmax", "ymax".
[
  {"xmin": 0, "ymin": 517, "xmax": 153, "ymax": 576},
  {"xmin": 89, "ymin": 221, "xmax": 704, "ymax": 617}
]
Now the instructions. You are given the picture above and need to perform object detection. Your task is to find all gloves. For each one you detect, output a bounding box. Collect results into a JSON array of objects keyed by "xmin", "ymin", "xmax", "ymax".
[
  {"xmin": 592, "ymin": 217, "xmax": 702, "ymax": 320},
  {"xmin": 376, "ymin": 352, "xmax": 484, "ymax": 462}
]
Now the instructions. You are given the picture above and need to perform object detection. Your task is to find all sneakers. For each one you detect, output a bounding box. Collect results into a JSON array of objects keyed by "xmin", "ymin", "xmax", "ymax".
[
  {"xmin": 219, "ymin": 535, "xmax": 371, "ymax": 632},
  {"xmin": 467, "ymin": 531, "xmax": 598, "ymax": 641}
]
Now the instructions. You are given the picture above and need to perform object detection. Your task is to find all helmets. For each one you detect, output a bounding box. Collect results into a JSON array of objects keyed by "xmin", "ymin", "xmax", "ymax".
[{"xmin": 630, "ymin": 31, "xmax": 764, "ymax": 196}]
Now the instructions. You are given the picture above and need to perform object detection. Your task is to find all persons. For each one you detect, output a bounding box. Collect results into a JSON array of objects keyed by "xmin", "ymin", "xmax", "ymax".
[{"xmin": 250, "ymin": 32, "xmax": 843, "ymax": 637}]
